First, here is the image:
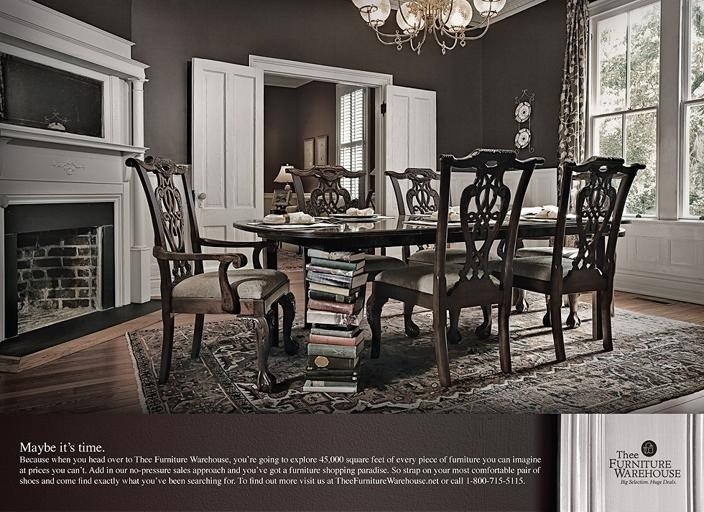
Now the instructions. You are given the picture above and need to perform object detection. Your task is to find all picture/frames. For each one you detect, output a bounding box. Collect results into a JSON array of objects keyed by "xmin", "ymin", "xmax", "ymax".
[
  {"xmin": 316, "ymin": 135, "xmax": 328, "ymax": 165},
  {"xmin": 302, "ymin": 137, "xmax": 315, "ymax": 171}
]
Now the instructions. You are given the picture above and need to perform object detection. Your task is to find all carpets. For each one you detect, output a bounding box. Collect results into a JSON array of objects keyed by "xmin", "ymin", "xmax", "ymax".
[{"xmin": 124, "ymin": 289, "xmax": 704, "ymax": 414}]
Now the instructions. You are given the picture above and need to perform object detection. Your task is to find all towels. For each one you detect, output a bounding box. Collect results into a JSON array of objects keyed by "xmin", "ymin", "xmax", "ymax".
[
  {"xmin": 263, "ymin": 211, "xmax": 316, "ymax": 224},
  {"xmin": 346, "ymin": 207, "xmax": 375, "ymax": 216},
  {"xmin": 431, "ymin": 209, "xmax": 460, "ymax": 221}
]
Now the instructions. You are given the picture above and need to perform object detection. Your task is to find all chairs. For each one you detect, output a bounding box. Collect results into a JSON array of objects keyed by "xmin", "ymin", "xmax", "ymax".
[
  {"xmin": 482, "ymin": 156, "xmax": 647, "ymax": 360},
  {"xmin": 516, "ymin": 172, "xmax": 627, "ymax": 328},
  {"xmin": 287, "ymin": 165, "xmax": 407, "ymax": 283},
  {"xmin": 366, "ymin": 147, "xmax": 544, "ymax": 387},
  {"xmin": 350, "ymin": 0, "xmax": 506, "ymax": 56},
  {"xmin": 124, "ymin": 154, "xmax": 299, "ymax": 392},
  {"xmin": 383, "ymin": 167, "xmax": 453, "ymax": 334}
]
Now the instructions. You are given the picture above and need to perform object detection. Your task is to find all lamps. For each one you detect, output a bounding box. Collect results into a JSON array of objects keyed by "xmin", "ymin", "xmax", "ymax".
[{"xmin": 273, "ymin": 163, "xmax": 295, "ymax": 206}]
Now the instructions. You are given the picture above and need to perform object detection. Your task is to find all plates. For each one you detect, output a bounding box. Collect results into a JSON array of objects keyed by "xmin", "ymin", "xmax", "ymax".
[
  {"xmin": 423, "ymin": 217, "xmax": 477, "ymax": 223},
  {"xmin": 523, "ymin": 215, "xmax": 557, "ymax": 220},
  {"xmin": 329, "ymin": 214, "xmax": 382, "ymax": 220},
  {"xmin": 256, "ymin": 219, "xmax": 317, "ymax": 229}
]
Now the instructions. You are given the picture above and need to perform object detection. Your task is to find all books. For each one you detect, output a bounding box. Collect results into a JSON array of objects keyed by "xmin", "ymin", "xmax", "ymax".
[{"xmin": 303, "ymin": 248, "xmax": 371, "ymax": 395}]
